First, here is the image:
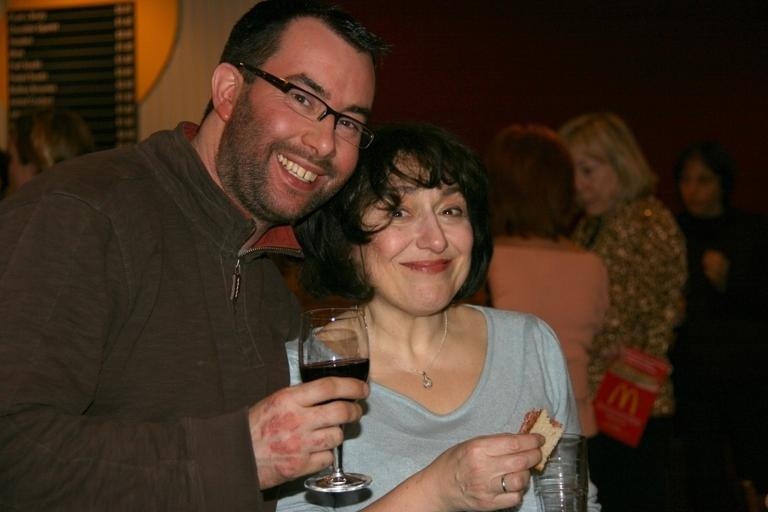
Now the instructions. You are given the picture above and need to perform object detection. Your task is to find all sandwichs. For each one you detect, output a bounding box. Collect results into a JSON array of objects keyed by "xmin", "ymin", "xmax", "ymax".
[{"xmin": 515, "ymin": 407, "xmax": 565, "ymax": 474}]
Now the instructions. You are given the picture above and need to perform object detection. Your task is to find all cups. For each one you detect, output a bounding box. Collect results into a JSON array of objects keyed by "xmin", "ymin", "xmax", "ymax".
[
  {"xmin": 532, "ymin": 433, "xmax": 588, "ymax": 512},
  {"xmin": 298, "ymin": 307, "xmax": 373, "ymax": 493}
]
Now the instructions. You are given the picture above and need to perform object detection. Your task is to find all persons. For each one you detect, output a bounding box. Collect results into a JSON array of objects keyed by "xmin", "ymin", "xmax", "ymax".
[
  {"xmin": 472, "ymin": 122, "xmax": 612, "ymax": 512},
  {"xmin": 0, "ymin": 2, "xmax": 378, "ymax": 512},
  {"xmin": 561, "ymin": 112, "xmax": 687, "ymax": 512},
  {"xmin": 31, "ymin": 110, "xmax": 105, "ymax": 171},
  {"xmin": 280, "ymin": 118, "xmax": 600, "ymax": 511},
  {"xmin": 665, "ymin": 139, "xmax": 768, "ymax": 510}
]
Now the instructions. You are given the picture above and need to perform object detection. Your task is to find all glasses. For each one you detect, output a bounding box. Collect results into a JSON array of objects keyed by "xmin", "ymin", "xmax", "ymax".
[{"xmin": 232, "ymin": 61, "xmax": 375, "ymax": 150}]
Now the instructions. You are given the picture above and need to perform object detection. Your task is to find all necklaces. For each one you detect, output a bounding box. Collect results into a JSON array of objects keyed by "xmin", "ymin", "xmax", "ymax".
[{"xmin": 363, "ymin": 315, "xmax": 447, "ymax": 391}]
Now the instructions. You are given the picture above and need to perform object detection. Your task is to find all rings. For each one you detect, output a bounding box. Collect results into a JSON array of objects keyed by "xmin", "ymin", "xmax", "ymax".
[{"xmin": 498, "ymin": 476, "xmax": 508, "ymax": 495}]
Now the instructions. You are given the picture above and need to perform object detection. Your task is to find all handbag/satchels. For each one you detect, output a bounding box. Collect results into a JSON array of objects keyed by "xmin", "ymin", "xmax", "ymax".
[{"xmin": 592, "ymin": 344, "xmax": 668, "ymax": 445}]
